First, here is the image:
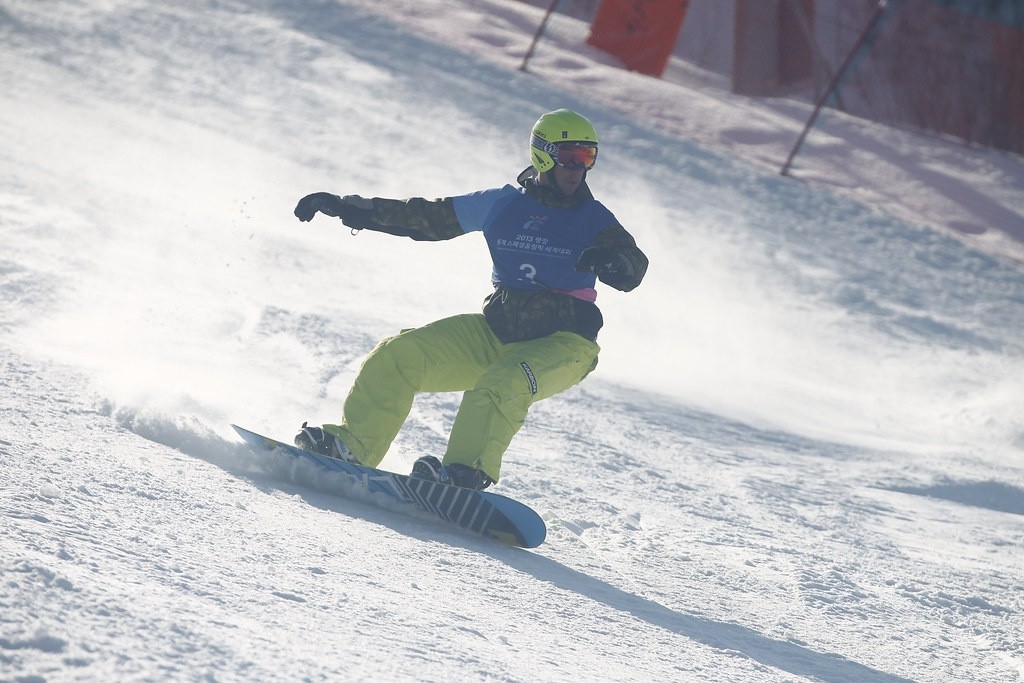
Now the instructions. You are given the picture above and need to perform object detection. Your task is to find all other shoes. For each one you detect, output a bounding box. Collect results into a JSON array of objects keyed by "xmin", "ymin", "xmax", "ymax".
[
  {"xmin": 411, "ymin": 455, "xmax": 485, "ymax": 491},
  {"xmin": 295, "ymin": 422, "xmax": 360, "ymax": 465}
]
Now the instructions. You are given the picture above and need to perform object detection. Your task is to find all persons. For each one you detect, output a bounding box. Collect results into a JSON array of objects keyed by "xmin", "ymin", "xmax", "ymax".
[{"xmin": 293, "ymin": 106, "xmax": 648, "ymax": 490}]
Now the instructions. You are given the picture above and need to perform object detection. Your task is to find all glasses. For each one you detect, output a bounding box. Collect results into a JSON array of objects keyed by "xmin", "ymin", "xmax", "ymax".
[{"xmin": 550, "ymin": 143, "xmax": 598, "ymax": 170}]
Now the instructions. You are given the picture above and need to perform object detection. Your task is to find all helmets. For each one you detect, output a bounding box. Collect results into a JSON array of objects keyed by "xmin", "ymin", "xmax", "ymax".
[{"xmin": 529, "ymin": 109, "xmax": 598, "ymax": 173}]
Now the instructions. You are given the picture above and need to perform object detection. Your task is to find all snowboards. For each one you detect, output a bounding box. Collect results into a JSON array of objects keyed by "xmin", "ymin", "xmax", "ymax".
[{"xmin": 231, "ymin": 423, "xmax": 547, "ymax": 550}]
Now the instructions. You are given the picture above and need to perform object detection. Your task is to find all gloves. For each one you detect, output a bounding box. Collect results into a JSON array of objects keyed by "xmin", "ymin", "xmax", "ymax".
[
  {"xmin": 294, "ymin": 191, "xmax": 373, "ymax": 229},
  {"xmin": 574, "ymin": 247, "xmax": 632, "ymax": 291}
]
